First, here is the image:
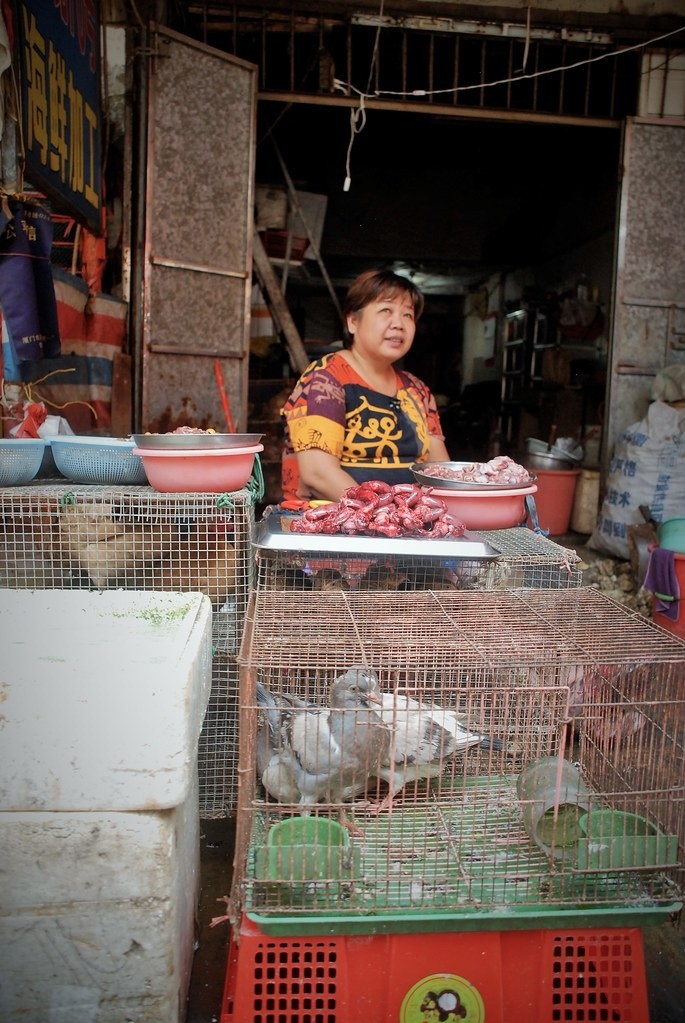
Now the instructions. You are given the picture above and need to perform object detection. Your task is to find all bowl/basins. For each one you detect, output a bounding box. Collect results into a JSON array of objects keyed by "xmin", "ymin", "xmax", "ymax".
[
  {"xmin": 658, "ymin": 519, "xmax": 685, "ymax": 554},
  {"xmin": 580, "ymin": 810, "xmax": 664, "ymax": 838},
  {"xmin": 269, "ymin": 816, "xmax": 347, "ymax": 846},
  {"xmin": 522, "ymin": 452, "xmax": 572, "ymax": 471},
  {"xmin": 527, "ymin": 438, "xmax": 580, "ymax": 461},
  {"xmin": 421, "ymin": 484, "xmax": 537, "ymax": 530},
  {"xmin": 133, "ymin": 442, "xmax": 264, "ymax": 492}
]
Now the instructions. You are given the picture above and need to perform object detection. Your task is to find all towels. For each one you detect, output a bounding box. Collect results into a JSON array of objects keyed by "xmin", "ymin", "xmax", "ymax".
[{"xmin": 641, "ymin": 548, "xmax": 682, "ymax": 624}]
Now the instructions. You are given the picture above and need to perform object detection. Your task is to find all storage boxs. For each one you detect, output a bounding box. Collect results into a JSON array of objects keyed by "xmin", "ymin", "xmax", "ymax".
[
  {"xmin": 1, "ymin": 757, "xmax": 201, "ymax": 1023},
  {"xmin": 0, "ymin": 588, "xmax": 212, "ymax": 812},
  {"xmin": 287, "ymin": 189, "xmax": 328, "ymax": 259}
]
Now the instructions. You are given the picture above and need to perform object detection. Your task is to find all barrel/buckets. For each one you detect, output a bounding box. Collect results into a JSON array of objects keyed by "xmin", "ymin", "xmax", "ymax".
[
  {"xmin": 653, "ymin": 552, "xmax": 685, "ymax": 640},
  {"xmin": 528, "ymin": 470, "xmax": 580, "ymax": 536}
]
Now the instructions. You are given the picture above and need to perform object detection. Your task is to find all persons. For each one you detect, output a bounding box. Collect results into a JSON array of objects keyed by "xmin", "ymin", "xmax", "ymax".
[{"xmin": 281, "ymin": 274, "xmax": 451, "ymax": 583}]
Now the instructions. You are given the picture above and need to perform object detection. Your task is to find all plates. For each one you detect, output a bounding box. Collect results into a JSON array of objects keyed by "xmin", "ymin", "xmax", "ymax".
[
  {"xmin": 129, "ymin": 434, "xmax": 266, "ymax": 448},
  {"xmin": 410, "ymin": 462, "xmax": 538, "ymax": 490}
]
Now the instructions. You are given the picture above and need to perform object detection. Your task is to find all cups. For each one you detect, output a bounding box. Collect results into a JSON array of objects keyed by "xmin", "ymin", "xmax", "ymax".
[{"xmin": 516, "ymin": 757, "xmax": 592, "ymax": 859}]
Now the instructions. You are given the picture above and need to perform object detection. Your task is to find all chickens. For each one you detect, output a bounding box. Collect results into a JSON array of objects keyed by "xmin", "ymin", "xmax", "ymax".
[
  {"xmin": 271, "ymin": 560, "xmax": 460, "ymax": 591},
  {"xmin": 20, "ymin": 502, "xmax": 242, "ymax": 608},
  {"xmin": 254, "ymin": 664, "xmax": 486, "ymax": 839}
]
